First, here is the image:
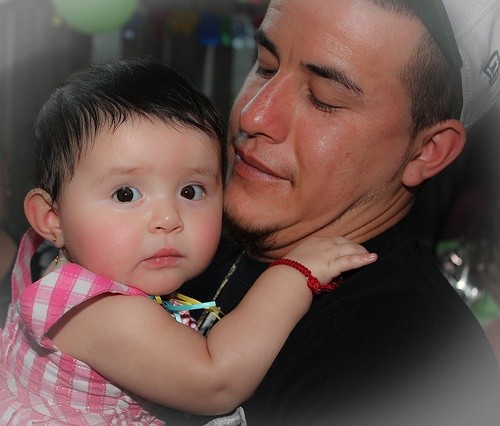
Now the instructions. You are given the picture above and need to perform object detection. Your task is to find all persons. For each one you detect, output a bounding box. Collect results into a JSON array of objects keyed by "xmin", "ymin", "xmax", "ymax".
[
  {"xmin": 1, "ymin": 1, "xmax": 500, "ymax": 426},
  {"xmin": 0, "ymin": 59, "xmax": 378, "ymax": 426}
]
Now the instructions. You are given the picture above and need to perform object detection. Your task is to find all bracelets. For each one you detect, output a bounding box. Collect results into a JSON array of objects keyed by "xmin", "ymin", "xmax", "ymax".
[{"xmin": 264, "ymin": 257, "xmax": 340, "ymax": 294}]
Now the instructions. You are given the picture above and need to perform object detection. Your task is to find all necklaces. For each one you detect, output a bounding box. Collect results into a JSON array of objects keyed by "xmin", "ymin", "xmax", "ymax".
[
  {"xmin": 195, "ymin": 243, "xmax": 251, "ymax": 337},
  {"xmin": 49, "ymin": 252, "xmax": 67, "ymax": 263}
]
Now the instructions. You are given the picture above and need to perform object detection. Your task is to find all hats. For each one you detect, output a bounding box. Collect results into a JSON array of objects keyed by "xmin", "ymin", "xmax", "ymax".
[{"xmin": 408, "ymin": 1, "xmax": 498, "ymax": 131}]
